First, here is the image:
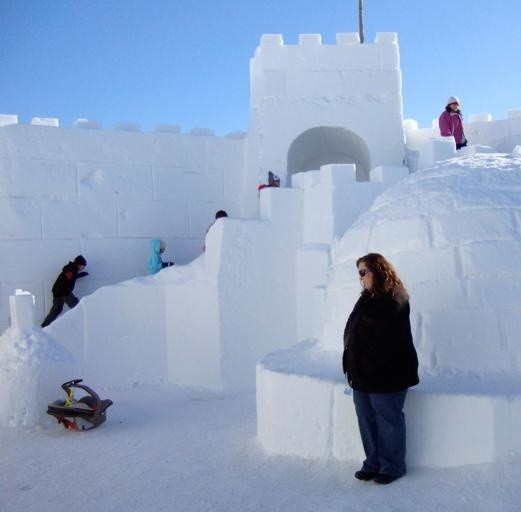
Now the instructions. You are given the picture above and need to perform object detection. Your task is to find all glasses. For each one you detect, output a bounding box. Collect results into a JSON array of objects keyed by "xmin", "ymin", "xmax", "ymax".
[{"xmin": 359, "ymin": 269, "xmax": 371, "ymax": 277}]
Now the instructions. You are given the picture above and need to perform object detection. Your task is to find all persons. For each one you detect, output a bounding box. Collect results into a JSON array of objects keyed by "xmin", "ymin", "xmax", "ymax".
[
  {"xmin": 438, "ymin": 96, "xmax": 468, "ymax": 151},
  {"xmin": 201, "ymin": 209, "xmax": 229, "ymax": 253},
  {"xmin": 257, "ymin": 170, "xmax": 281, "ymax": 193},
  {"xmin": 147, "ymin": 239, "xmax": 174, "ymax": 276},
  {"xmin": 341, "ymin": 253, "xmax": 420, "ymax": 485},
  {"xmin": 40, "ymin": 254, "xmax": 90, "ymax": 328}
]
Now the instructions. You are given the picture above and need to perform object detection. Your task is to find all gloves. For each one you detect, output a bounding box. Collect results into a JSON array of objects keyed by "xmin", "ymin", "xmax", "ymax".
[{"xmin": 79, "ymin": 272, "xmax": 88, "ymax": 277}]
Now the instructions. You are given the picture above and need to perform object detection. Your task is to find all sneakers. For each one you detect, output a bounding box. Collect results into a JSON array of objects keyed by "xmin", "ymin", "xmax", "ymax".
[{"xmin": 355, "ymin": 471, "xmax": 400, "ymax": 484}]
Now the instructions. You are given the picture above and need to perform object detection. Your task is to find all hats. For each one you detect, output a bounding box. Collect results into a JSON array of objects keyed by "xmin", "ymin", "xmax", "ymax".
[
  {"xmin": 216, "ymin": 211, "xmax": 227, "ymax": 219},
  {"xmin": 269, "ymin": 172, "xmax": 280, "ymax": 184},
  {"xmin": 448, "ymin": 96, "xmax": 459, "ymax": 106},
  {"xmin": 75, "ymin": 255, "xmax": 86, "ymax": 266},
  {"xmin": 160, "ymin": 242, "xmax": 166, "ymax": 250}
]
